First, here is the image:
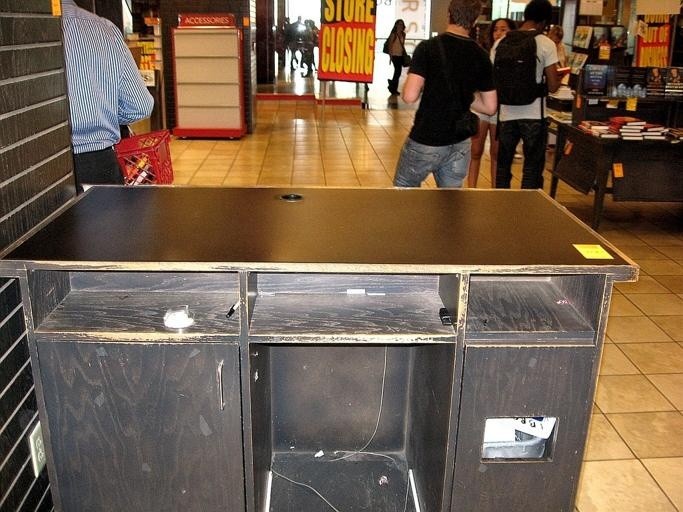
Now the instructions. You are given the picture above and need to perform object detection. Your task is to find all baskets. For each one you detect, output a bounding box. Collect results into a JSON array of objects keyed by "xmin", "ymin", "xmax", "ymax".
[{"xmin": 116, "ymin": 129, "xmax": 174, "ymax": 187}]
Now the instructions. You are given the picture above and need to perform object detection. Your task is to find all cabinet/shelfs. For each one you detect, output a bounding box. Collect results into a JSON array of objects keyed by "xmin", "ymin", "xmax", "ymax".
[{"xmin": 0, "ymin": 183, "xmax": 642, "ymax": 512}]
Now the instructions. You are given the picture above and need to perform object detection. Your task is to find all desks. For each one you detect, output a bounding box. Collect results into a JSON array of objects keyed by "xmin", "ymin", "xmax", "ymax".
[{"xmin": 550, "ymin": 122, "xmax": 683, "ymax": 221}]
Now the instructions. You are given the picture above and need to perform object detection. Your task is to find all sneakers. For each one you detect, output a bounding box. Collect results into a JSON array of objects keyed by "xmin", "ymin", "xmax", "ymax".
[{"xmin": 388, "ymin": 78, "xmax": 399, "ymax": 96}]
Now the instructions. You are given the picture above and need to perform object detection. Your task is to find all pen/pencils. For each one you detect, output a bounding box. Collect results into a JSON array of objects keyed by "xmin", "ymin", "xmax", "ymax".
[{"xmin": 226, "ymin": 301, "xmax": 240, "ymax": 318}]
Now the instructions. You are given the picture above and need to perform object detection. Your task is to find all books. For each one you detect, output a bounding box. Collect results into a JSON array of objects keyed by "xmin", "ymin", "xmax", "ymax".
[
  {"xmin": 583, "ymin": 64, "xmax": 683, "ymax": 98},
  {"xmin": 576, "ymin": 116, "xmax": 683, "ymax": 144},
  {"xmin": 557, "ymin": 67, "xmax": 572, "ymax": 75}
]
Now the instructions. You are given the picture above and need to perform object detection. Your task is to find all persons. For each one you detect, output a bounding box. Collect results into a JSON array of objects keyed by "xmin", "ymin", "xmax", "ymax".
[
  {"xmin": 388, "ymin": 19, "xmax": 406, "ymax": 96},
  {"xmin": 283, "ymin": 16, "xmax": 320, "ymax": 78},
  {"xmin": 60, "ymin": 0, "xmax": 155, "ymax": 195},
  {"xmin": 393, "ymin": 0, "xmax": 567, "ymax": 188}
]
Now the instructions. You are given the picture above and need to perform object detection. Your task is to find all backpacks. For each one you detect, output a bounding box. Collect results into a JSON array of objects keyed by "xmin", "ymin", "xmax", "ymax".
[{"xmin": 491, "ymin": 28, "xmax": 550, "ymax": 108}]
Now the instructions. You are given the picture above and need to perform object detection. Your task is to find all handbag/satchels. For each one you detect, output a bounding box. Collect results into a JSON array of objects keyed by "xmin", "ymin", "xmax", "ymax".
[{"xmin": 401, "ymin": 52, "xmax": 412, "ymax": 69}]
